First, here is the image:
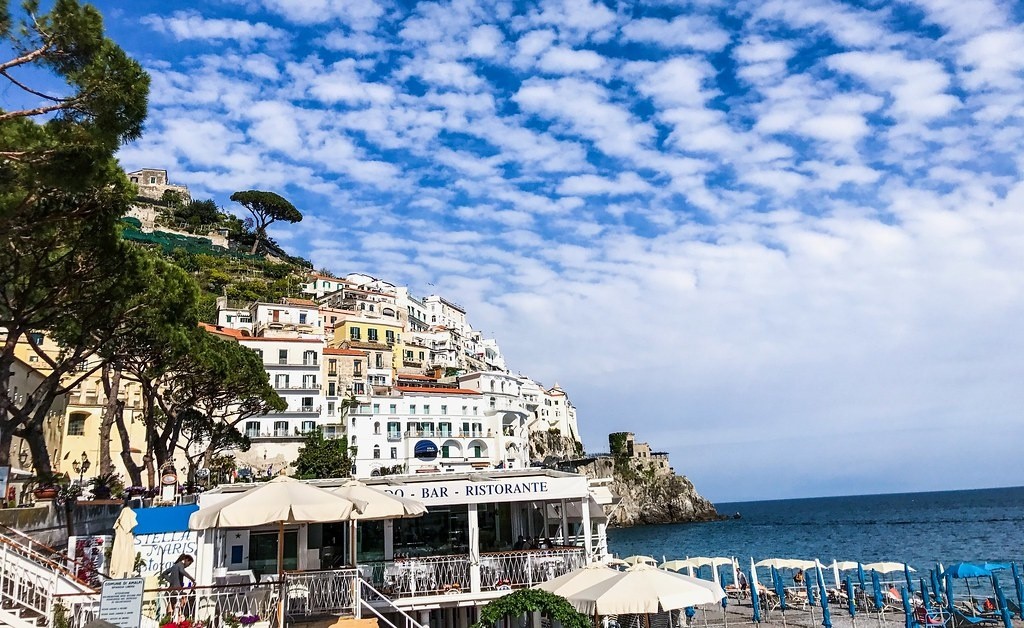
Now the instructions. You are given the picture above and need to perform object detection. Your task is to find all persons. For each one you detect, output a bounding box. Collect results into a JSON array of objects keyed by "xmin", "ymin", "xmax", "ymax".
[
  {"xmin": 155, "ymin": 552, "xmax": 195, "ymax": 621},
  {"xmin": 888, "ymin": 583, "xmax": 901, "ymax": 604},
  {"xmin": 735, "ymin": 566, "xmax": 748, "ymax": 599},
  {"xmin": 794, "ymin": 568, "xmax": 806, "ymax": 591},
  {"xmin": 513, "ymin": 534, "xmax": 554, "ymax": 581}
]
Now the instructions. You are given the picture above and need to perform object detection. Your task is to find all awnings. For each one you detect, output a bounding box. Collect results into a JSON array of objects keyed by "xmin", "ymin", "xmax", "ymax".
[
  {"xmin": 415, "ymin": 440, "xmax": 438, "ymax": 454},
  {"xmin": 534, "ymin": 493, "xmax": 609, "ymax": 525}
]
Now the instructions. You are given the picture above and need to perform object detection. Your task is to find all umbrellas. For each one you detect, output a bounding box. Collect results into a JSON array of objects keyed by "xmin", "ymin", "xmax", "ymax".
[
  {"xmin": 526, "ymin": 556, "xmax": 1024, "ymax": 628},
  {"xmin": 183, "ymin": 475, "xmax": 368, "ymax": 628},
  {"xmin": 332, "ymin": 480, "xmax": 428, "ymax": 607}
]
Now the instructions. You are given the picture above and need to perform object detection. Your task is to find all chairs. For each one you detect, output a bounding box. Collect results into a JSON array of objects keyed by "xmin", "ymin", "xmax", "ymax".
[
  {"xmin": 954, "ymin": 608, "xmax": 999, "ymax": 628},
  {"xmin": 1006, "ymin": 598, "xmax": 1021, "ymax": 614},
  {"xmin": 973, "ymin": 602, "xmax": 1003, "ymax": 620},
  {"xmin": 213, "ymin": 565, "xmax": 375, "ymax": 616},
  {"xmin": 962, "ymin": 601, "xmax": 995, "ymax": 622},
  {"xmin": 988, "ymin": 598, "xmax": 1001, "ymax": 610},
  {"xmin": 723, "ymin": 582, "xmax": 987, "ymax": 628},
  {"xmin": 383, "ymin": 556, "xmax": 504, "ymax": 598},
  {"xmin": 525, "ymin": 556, "xmax": 565, "ymax": 582}
]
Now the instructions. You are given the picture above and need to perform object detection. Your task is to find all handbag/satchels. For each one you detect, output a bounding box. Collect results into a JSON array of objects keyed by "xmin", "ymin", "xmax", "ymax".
[{"xmin": 184, "ymin": 590, "xmax": 196, "ymax": 617}]
